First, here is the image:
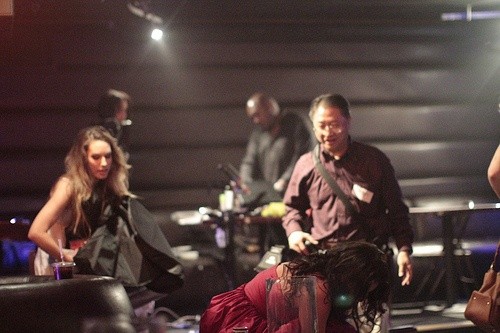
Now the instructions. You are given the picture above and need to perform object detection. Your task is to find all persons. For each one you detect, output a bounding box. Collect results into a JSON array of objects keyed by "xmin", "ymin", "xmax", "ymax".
[
  {"xmin": 27, "ymin": 126, "xmax": 141, "ymax": 277},
  {"xmin": 238, "ymin": 92, "xmax": 317, "ymax": 199},
  {"xmin": 97, "ymin": 89, "xmax": 134, "ymax": 161},
  {"xmin": 487, "ymin": 143, "xmax": 499, "ymax": 199},
  {"xmin": 199, "ymin": 240, "xmax": 392, "ymax": 333},
  {"xmin": 282, "ymin": 93, "xmax": 415, "ymax": 333}
]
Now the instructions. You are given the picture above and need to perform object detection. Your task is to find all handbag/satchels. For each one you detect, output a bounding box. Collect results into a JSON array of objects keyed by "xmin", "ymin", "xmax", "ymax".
[
  {"xmin": 369, "ymin": 240, "xmax": 397, "ymax": 288},
  {"xmin": 463, "ymin": 240, "xmax": 500, "ymax": 333},
  {"xmin": 73, "ymin": 194, "xmax": 184, "ymax": 304}
]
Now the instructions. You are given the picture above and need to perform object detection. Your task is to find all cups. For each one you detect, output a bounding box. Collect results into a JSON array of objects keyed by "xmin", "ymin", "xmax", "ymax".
[{"xmin": 51, "ymin": 262, "xmax": 76, "ymax": 281}]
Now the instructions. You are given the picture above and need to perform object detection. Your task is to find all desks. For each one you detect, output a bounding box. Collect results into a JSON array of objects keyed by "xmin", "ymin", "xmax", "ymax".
[
  {"xmin": 407, "ymin": 201, "xmax": 500, "ymax": 301},
  {"xmin": 203, "ymin": 216, "xmax": 282, "ymax": 286}
]
